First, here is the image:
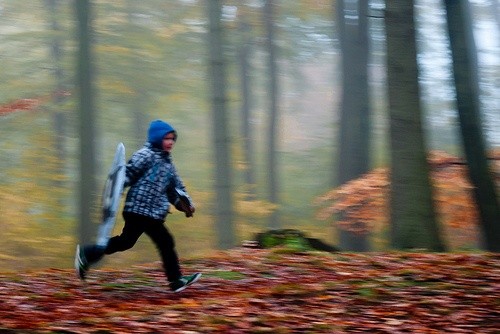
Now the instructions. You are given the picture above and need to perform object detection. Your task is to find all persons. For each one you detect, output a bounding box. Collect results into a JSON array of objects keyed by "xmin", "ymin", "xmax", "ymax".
[{"xmin": 74, "ymin": 118, "xmax": 202, "ymax": 294}]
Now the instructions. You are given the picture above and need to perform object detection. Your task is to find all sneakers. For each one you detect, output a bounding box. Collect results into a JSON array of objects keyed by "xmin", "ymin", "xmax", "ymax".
[
  {"xmin": 169, "ymin": 272, "xmax": 202, "ymax": 294},
  {"xmin": 74, "ymin": 243, "xmax": 89, "ymax": 281}
]
